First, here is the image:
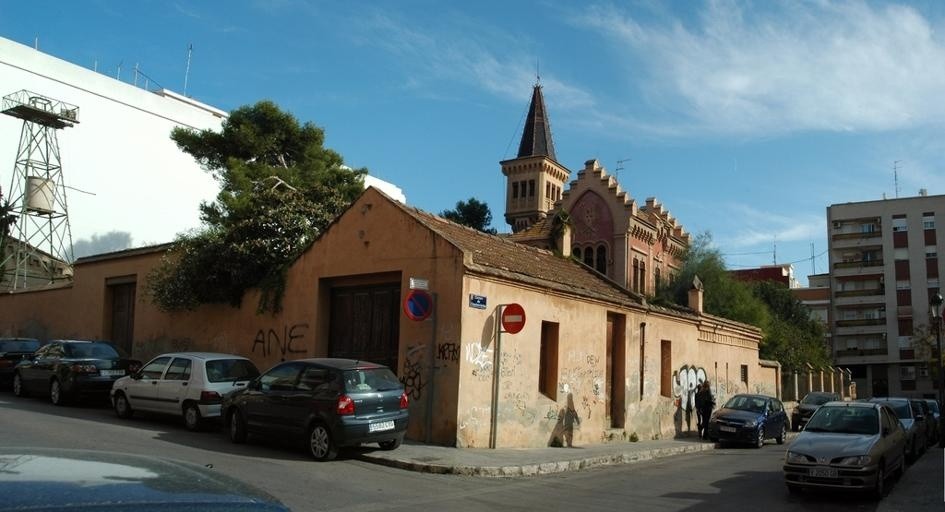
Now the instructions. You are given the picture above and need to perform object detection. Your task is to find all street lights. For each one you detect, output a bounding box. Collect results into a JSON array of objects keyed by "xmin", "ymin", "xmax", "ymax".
[{"xmin": 929, "ymin": 289, "xmax": 945, "ymax": 449}]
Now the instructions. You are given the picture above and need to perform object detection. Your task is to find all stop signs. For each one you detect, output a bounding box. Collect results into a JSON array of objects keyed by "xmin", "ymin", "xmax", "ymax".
[
  {"xmin": 403, "ymin": 288, "xmax": 434, "ymax": 322},
  {"xmin": 500, "ymin": 303, "xmax": 527, "ymax": 335}
]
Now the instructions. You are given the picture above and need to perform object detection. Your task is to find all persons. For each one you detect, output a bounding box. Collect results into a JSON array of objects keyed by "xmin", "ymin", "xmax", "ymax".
[
  {"xmin": 695, "ymin": 384, "xmax": 705, "ymax": 438},
  {"xmin": 700, "ymin": 380, "xmax": 716, "ymax": 439}
]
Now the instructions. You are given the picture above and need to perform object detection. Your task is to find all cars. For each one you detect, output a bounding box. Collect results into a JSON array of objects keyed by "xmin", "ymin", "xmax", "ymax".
[
  {"xmin": 12, "ymin": 338, "xmax": 144, "ymax": 409},
  {"xmin": 218, "ymin": 355, "xmax": 412, "ymax": 460},
  {"xmin": 790, "ymin": 388, "xmax": 941, "ymax": 460},
  {"xmin": 781, "ymin": 398, "xmax": 908, "ymax": 501},
  {"xmin": 0, "ymin": 441, "xmax": 294, "ymax": 511},
  {"xmin": 709, "ymin": 392, "xmax": 790, "ymax": 450},
  {"xmin": 107, "ymin": 349, "xmax": 262, "ymax": 432},
  {"xmin": 0, "ymin": 335, "xmax": 45, "ymax": 375}
]
